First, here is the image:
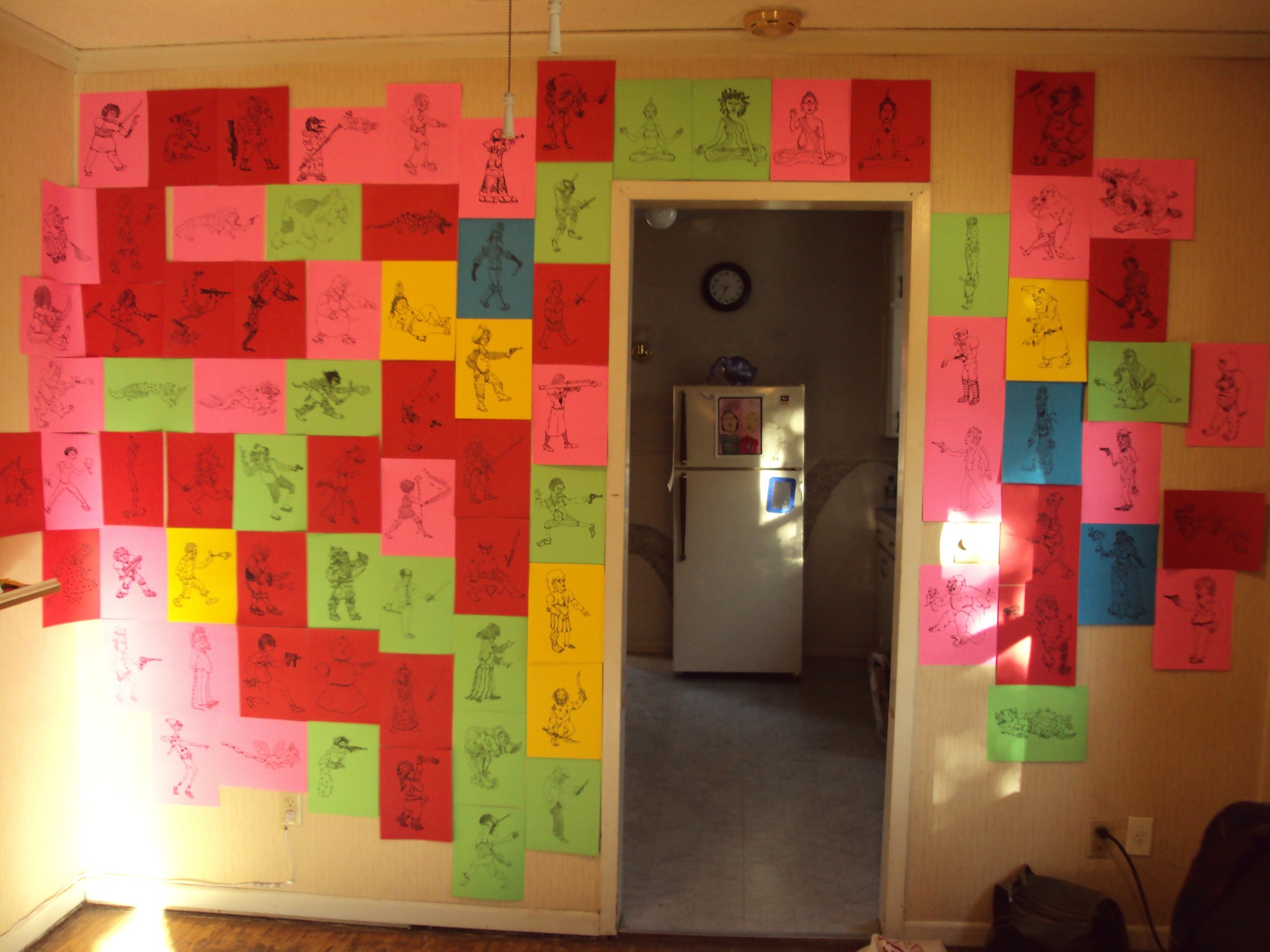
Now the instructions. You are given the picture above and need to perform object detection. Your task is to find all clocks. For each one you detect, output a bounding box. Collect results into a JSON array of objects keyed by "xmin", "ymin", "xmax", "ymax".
[{"xmin": 703, "ymin": 262, "xmax": 751, "ymax": 310}]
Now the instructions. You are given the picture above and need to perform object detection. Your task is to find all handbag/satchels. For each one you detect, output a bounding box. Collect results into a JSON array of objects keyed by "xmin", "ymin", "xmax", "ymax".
[{"xmin": 991, "ymin": 864, "xmax": 1129, "ymax": 952}]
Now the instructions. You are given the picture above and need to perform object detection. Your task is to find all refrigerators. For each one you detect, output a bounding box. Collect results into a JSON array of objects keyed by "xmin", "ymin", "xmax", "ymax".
[{"xmin": 671, "ymin": 383, "xmax": 807, "ymax": 675}]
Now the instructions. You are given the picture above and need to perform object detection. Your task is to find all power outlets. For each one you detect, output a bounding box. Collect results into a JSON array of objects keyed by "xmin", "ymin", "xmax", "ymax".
[
  {"xmin": 1086, "ymin": 821, "xmax": 1116, "ymax": 858},
  {"xmin": 1125, "ymin": 817, "xmax": 1153, "ymax": 857}
]
